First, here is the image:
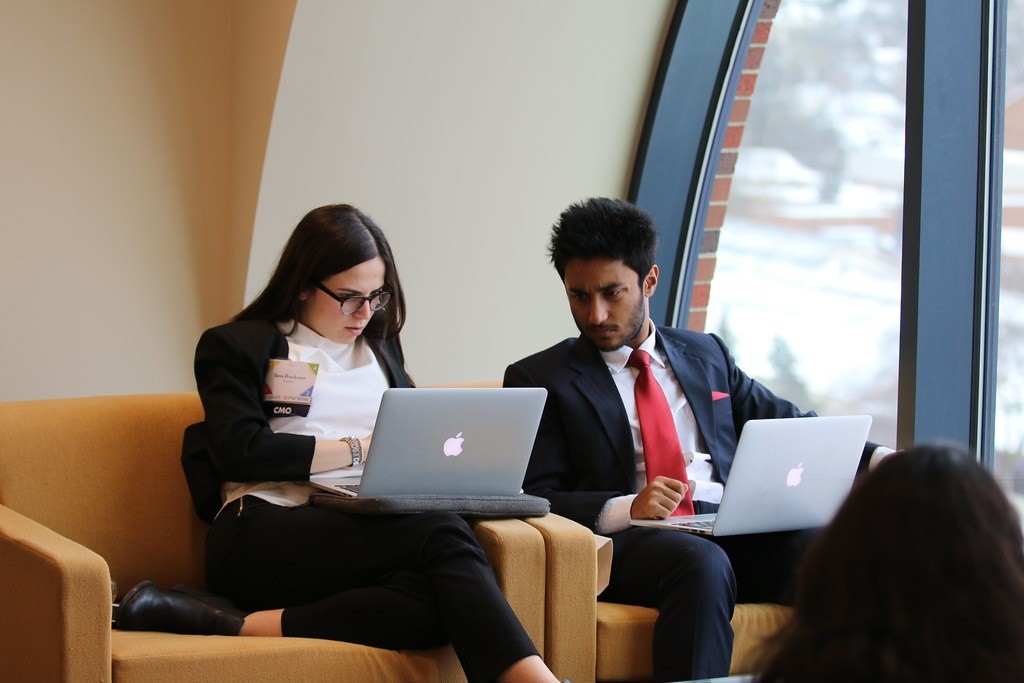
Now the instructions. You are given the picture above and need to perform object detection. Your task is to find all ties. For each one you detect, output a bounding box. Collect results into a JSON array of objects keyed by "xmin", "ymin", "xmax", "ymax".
[{"xmin": 628, "ymin": 350, "xmax": 695, "ymax": 516}]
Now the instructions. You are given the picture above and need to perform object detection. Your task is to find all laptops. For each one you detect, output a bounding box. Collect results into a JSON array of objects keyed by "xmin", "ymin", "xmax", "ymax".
[
  {"xmin": 304, "ymin": 386, "xmax": 548, "ymax": 499},
  {"xmin": 627, "ymin": 414, "xmax": 872, "ymax": 536}
]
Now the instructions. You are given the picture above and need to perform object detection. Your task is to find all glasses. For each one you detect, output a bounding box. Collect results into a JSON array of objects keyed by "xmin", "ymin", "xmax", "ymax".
[{"xmin": 312, "ymin": 280, "xmax": 395, "ymax": 316}]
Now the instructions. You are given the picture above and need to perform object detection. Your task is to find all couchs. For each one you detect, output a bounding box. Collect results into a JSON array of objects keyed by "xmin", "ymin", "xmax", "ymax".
[{"xmin": 0, "ymin": 382, "xmax": 801, "ymax": 683}]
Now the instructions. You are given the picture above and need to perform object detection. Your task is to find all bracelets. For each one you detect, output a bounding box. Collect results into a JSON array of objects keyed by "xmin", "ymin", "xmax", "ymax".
[{"xmin": 339, "ymin": 436, "xmax": 359, "ymax": 467}]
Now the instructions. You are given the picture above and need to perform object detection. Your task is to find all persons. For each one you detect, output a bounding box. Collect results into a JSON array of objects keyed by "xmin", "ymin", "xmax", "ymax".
[
  {"xmin": 116, "ymin": 204, "xmax": 573, "ymax": 683},
  {"xmin": 500, "ymin": 195, "xmax": 904, "ymax": 683},
  {"xmin": 751, "ymin": 444, "xmax": 1024, "ymax": 683}
]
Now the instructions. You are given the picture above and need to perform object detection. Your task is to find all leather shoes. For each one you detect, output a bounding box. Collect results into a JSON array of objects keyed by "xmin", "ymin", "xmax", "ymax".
[{"xmin": 115, "ymin": 580, "xmax": 253, "ymax": 636}]
